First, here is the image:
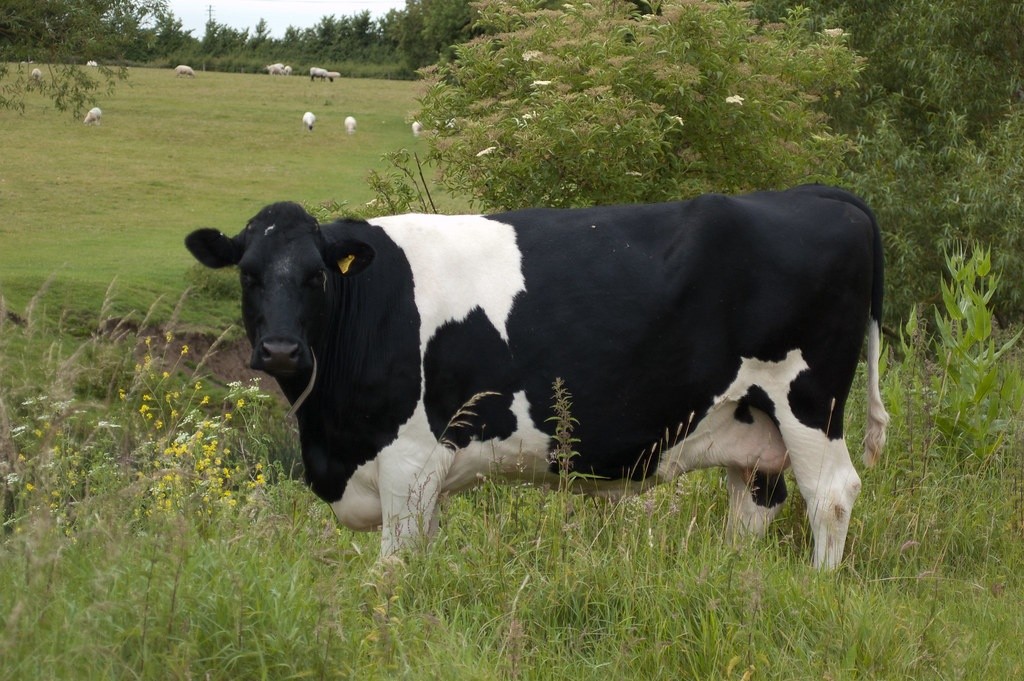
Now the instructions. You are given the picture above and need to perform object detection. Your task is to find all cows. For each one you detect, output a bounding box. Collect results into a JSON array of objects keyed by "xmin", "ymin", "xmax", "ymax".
[{"xmin": 183, "ymin": 183, "xmax": 891, "ymax": 569}]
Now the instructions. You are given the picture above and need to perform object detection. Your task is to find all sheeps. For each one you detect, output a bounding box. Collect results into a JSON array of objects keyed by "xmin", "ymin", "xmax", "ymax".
[
  {"xmin": 173, "ymin": 63, "xmax": 342, "ymax": 83},
  {"xmin": 32, "ymin": 60, "xmax": 102, "ymax": 126},
  {"xmin": 302, "ymin": 112, "xmax": 316, "ymax": 131},
  {"xmin": 344, "ymin": 116, "xmax": 357, "ymax": 135}
]
